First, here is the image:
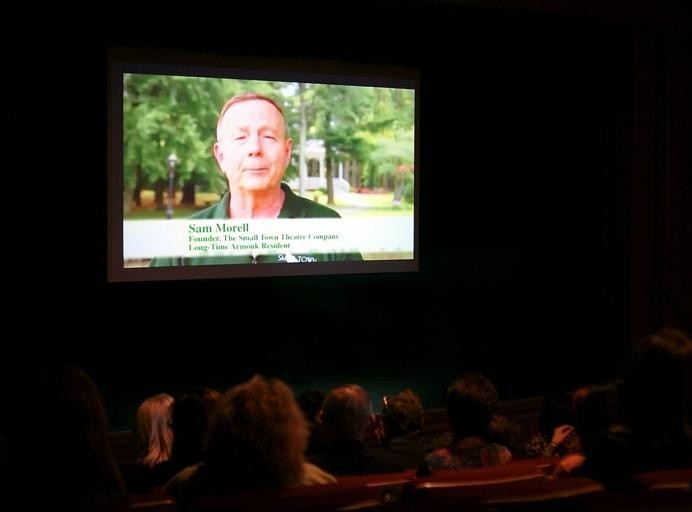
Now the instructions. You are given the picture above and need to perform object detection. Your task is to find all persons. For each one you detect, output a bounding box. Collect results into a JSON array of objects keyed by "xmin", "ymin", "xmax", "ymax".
[
  {"xmin": 0, "ymin": 319, "xmax": 692, "ymax": 512},
  {"xmin": 150, "ymin": 95, "xmax": 366, "ymax": 269}
]
